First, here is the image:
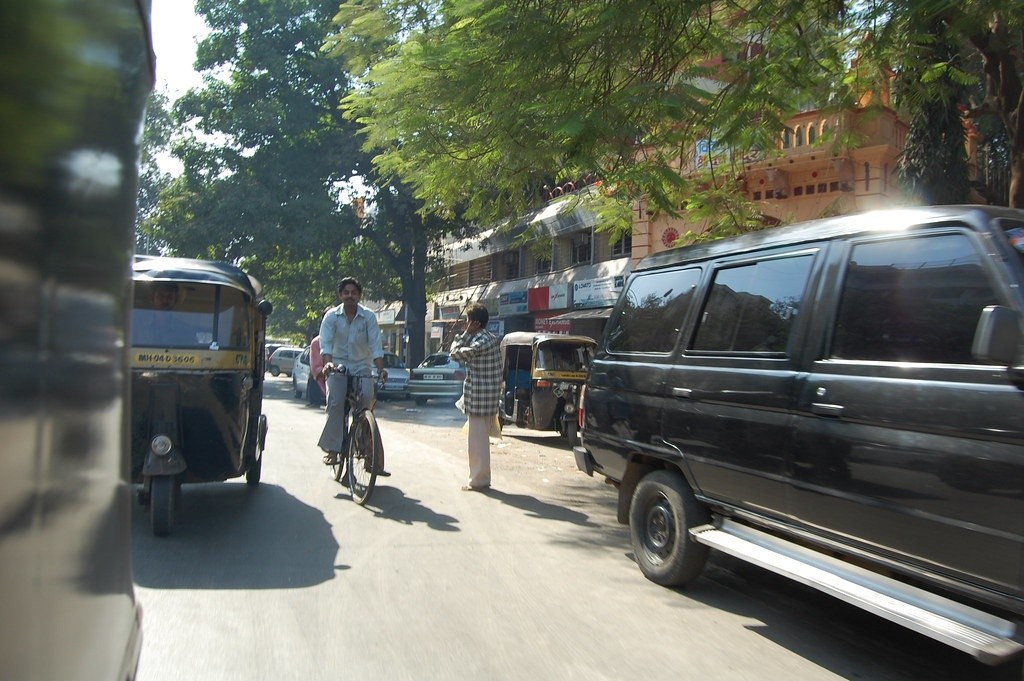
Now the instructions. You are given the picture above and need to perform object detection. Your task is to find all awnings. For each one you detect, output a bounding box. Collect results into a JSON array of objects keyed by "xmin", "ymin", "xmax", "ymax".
[
  {"xmin": 427, "ymin": 319, "xmax": 466, "ymax": 323},
  {"xmin": 546, "ymin": 307, "xmax": 614, "ymax": 320},
  {"xmin": 422, "ymin": 185, "xmax": 620, "ymax": 275}
]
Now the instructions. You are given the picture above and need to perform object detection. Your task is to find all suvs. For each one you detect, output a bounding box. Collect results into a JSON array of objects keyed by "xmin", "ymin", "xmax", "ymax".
[{"xmin": 575, "ymin": 205, "xmax": 1023, "ymax": 671}]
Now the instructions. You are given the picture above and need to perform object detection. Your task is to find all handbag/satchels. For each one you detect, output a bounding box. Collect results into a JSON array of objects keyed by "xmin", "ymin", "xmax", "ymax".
[{"xmin": 462, "ymin": 409, "xmax": 502, "ymax": 438}]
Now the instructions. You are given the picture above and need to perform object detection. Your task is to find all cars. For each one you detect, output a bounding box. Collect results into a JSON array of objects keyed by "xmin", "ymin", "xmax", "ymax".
[
  {"xmin": 292, "ymin": 345, "xmax": 319, "ymax": 400},
  {"xmin": 266, "ymin": 346, "xmax": 305, "ymax": 378},
  {"xmin": 264, "ymin": 338, "xmax": 290, "ymax": 365},
  {"xmin": 411, "ymin": 351, "xmax": 470, "ymax": 408},
  {"xmin": 374, "ymin": 350, "xmax": 411, "ymax": 401}
]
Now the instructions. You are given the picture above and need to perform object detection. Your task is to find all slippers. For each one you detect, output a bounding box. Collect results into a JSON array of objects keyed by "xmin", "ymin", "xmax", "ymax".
[
  {"xmin": 324, "ymin": 455, "xmax": 337, "ymax": 465},
  {"xmin": 366, "ymin": 467, "xmax": 392, "ymax": 476}
]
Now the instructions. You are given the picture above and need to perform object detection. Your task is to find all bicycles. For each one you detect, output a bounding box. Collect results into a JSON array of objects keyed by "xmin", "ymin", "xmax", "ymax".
[{"xmin": 319, "ymin": 363, "xmax": 391, "ymax": 506}]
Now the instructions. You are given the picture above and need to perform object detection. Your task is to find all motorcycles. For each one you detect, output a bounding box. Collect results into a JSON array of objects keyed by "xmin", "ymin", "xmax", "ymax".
[
  {"xmin": 498, "ymin": 332, "xmax": 599, "ymax": 449},
  {"xmin": 128, "ymin": 256, "xmax": 271, "ymax": 538}
]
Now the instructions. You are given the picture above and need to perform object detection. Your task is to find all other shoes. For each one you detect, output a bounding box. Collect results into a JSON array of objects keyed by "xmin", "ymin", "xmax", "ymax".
[{"xmin": 461, "ymin": 484, "xmax": 489, "ymax": 491}]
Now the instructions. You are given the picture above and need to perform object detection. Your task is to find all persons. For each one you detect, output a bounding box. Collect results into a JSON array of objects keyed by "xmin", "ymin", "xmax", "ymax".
[
  {"xmin": 557, "ymin": 349, "xmax": 585, "ymax": 371},
  {"xmin": 132, "ymin": 281, "xmax": 199, "ymax": 347},
  {"xmin": 309, "ymin": 277, "xmax": 392, "ymax": 477},
  {"xmin": 449, "ymin": 302, "xmax": 504, "ymax": 491}
]
